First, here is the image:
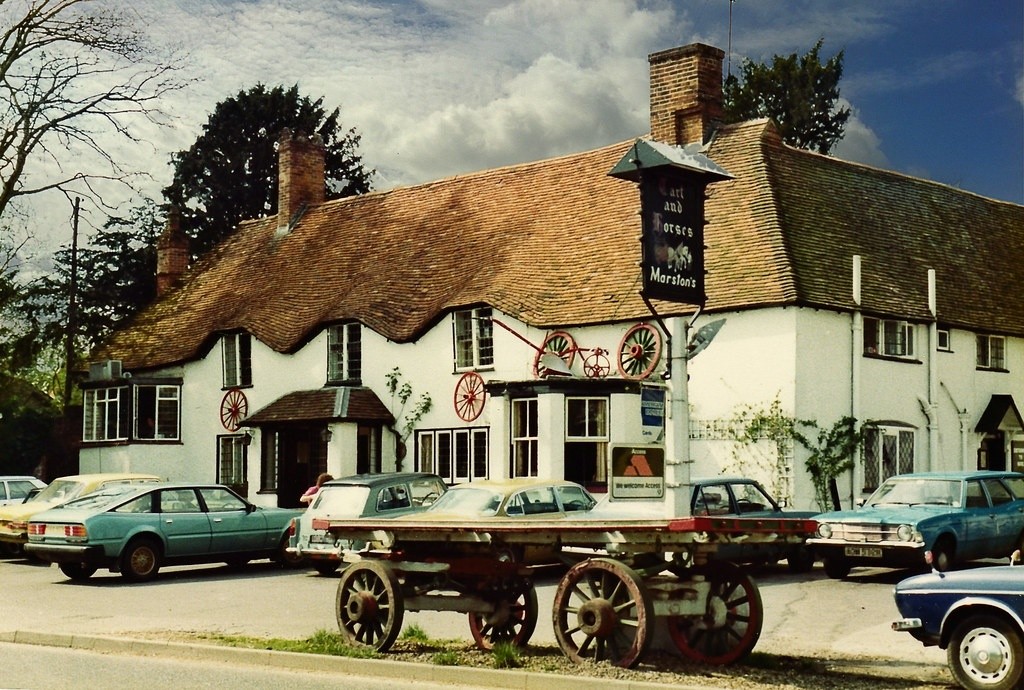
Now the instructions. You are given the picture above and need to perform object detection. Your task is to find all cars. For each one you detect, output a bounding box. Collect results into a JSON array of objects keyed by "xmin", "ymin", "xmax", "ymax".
[
  {"xmin": 0, "ymin": 476, "xmax": 48, "ymax": 510},
  {"xmin": 802, "ymin": 471, "xmax": 1023, "ymax": 580},
  {"xmin": 0, "ymin": 473, "xmax": 242, "ymax": 567},
  {"xmin": 589, "ymin": 476, "xmax": 826, "ymax": 576},
  {"xmin": 22, "ymin": 482, "xmax": 306, "ymax": 583},
  {"xmin": 891, "ymin": 547, "xmax": 1023, "ymax": 690},
  {"xmin": 423, "ymin": 479, "xmax": 600, "ymax": 519},
  {"xmin": 285, "ymin": 473, "xmax": 449, "ymax": 574}
]
